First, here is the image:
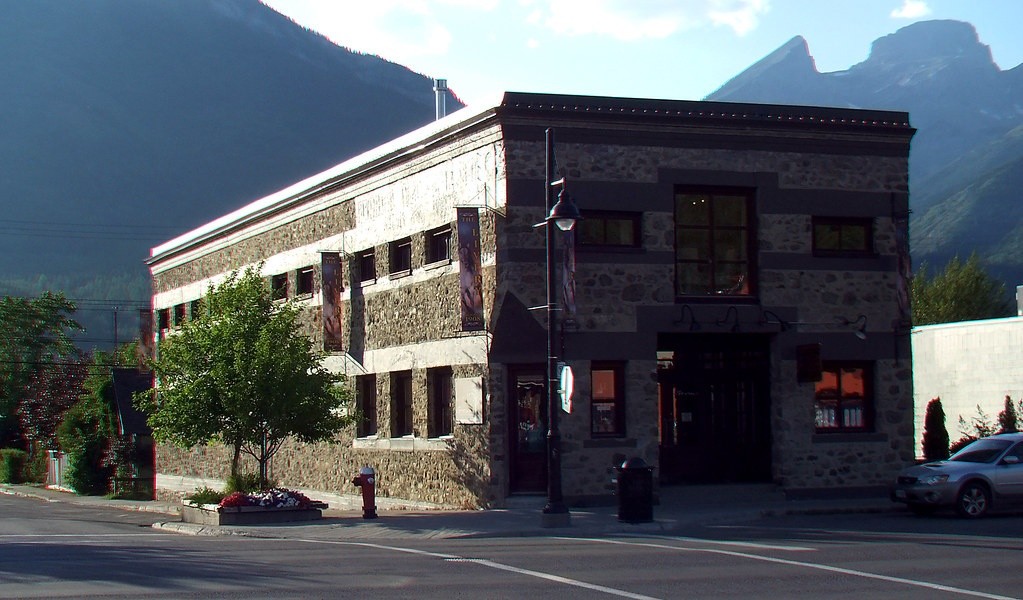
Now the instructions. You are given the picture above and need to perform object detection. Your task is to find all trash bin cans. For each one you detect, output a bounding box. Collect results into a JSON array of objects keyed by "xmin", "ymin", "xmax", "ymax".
[{"xmin": 612, "ymin": 457, "xmax": 659, "ymax": 524}]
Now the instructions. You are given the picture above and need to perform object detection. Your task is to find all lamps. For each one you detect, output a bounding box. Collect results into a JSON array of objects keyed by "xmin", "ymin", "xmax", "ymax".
[{"xmin": 671, "ymin": 304, "xmax": 868, "ymax": 340}]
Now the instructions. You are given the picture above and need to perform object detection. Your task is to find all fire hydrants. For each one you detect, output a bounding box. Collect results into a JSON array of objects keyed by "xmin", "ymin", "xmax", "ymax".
[{"xmin": 350, "ymin": 462, "xmax": 381, "ymax": 520}]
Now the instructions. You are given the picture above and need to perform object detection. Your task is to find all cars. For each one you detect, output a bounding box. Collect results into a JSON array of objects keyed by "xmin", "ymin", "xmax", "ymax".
[{"xmin": 887, "ymin": 429, "xmax": 1023, "ymax": 519}]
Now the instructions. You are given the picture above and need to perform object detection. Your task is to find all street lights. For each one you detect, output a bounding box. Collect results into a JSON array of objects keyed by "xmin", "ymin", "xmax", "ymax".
[
  {"xmin": 110, "ymin": 305, "xmax": 118, "ymax": 369},
  {"xmin": 526, "ymin": 127, "xmax": 586, "ymax": 514}
]
